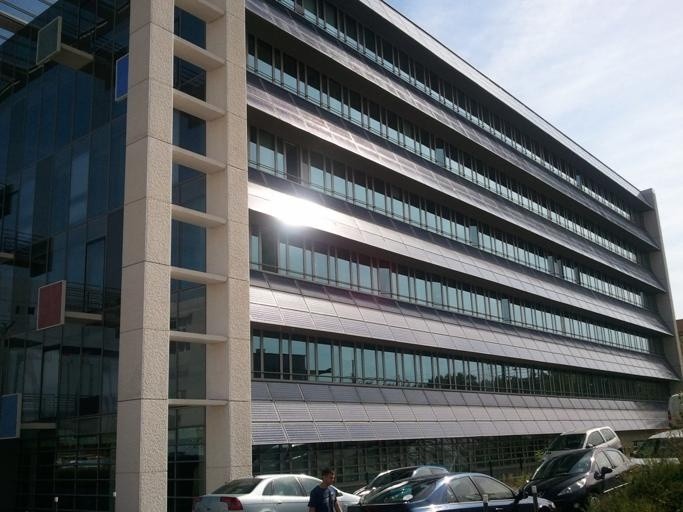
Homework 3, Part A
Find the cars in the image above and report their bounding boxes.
[192,473,361,512]
[542,425,624,461]
[352,465,448,496]
[513,448,645,512]
[632,426,682,472]
[347,471,558,512]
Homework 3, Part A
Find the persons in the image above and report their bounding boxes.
[308,467,343,512]
